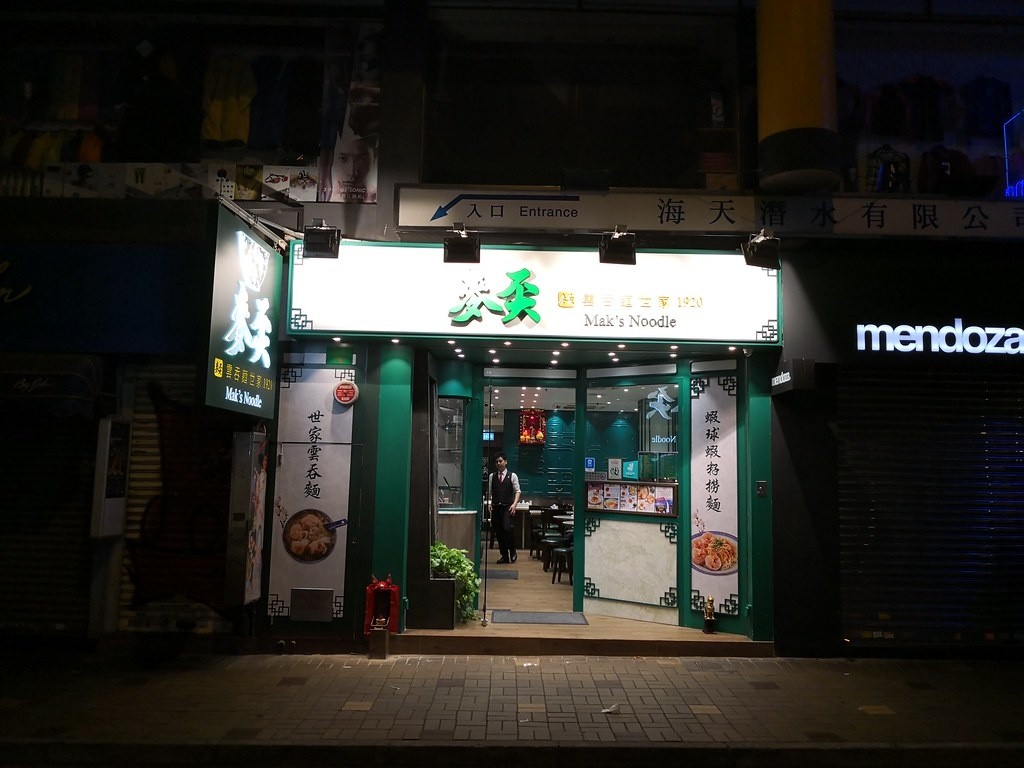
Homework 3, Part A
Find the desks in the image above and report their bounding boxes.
[551,514,575,538]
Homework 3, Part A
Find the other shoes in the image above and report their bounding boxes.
[497,555,509,563]
[510,549,517,562]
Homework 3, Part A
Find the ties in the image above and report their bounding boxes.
[499,472,504,483]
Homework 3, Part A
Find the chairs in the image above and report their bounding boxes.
[527,512,561,563]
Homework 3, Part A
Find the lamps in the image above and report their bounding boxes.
[302,218,342,257]
[598,225,637,265]
[739,228,783,271]
[442,222,481,264]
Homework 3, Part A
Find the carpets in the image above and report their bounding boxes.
[480,569,519,579]
[491,610,589,625]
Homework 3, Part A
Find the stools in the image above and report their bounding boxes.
[543,539,573,586]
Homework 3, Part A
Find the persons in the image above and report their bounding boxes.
[488,453,520,563]
[253,453,264,595]
[332,138,379,205]
